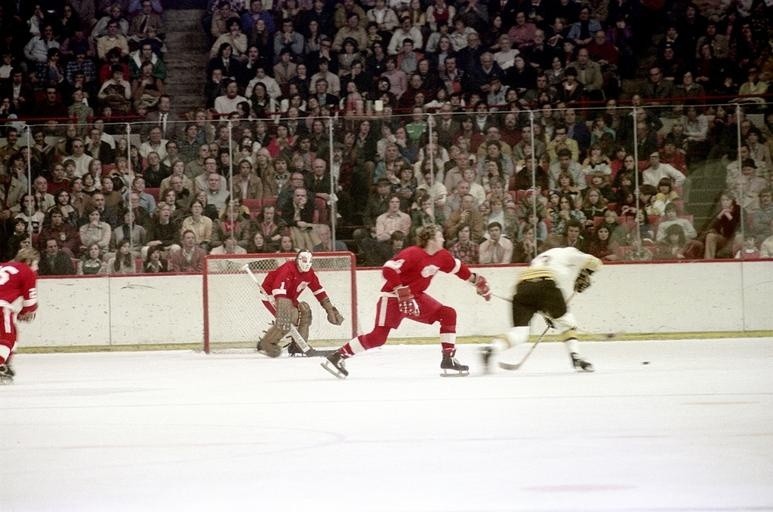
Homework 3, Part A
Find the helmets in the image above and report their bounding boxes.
[14,246,41,265]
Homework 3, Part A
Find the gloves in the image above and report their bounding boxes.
[470,273,491,302]
[574,269,590,293]
[394,285,420,316]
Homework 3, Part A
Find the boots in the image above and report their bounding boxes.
[480,343,492,369]
[440,348,468,371]
[0,363,14,378]
[570,352,592,369]
[325,349,348,376]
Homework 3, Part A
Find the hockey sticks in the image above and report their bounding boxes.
[240,264,337,356]
[497,289,576,369]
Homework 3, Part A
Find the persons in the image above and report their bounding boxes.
[478,245,606,372]
[327,222,492,377]
[252,247,344,357]
[0,0,772,274]
[0,247,41,377]
